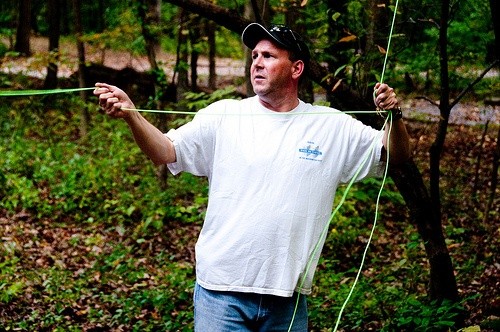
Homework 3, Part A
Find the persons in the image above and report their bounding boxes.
[93,22,409,331]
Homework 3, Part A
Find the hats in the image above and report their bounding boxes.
[241,23,310,63]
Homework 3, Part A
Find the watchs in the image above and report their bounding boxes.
[377,106,403,121]
